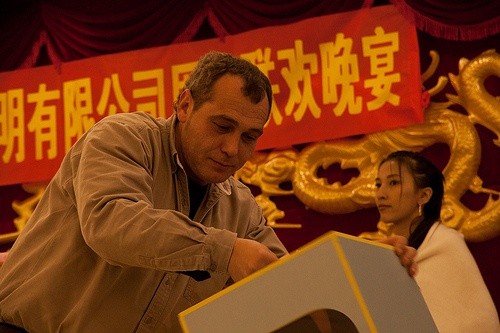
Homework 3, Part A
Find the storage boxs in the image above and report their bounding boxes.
[177,230,440,333]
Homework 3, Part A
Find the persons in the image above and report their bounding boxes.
[371,151,500,333]
[1,51,419,333]
